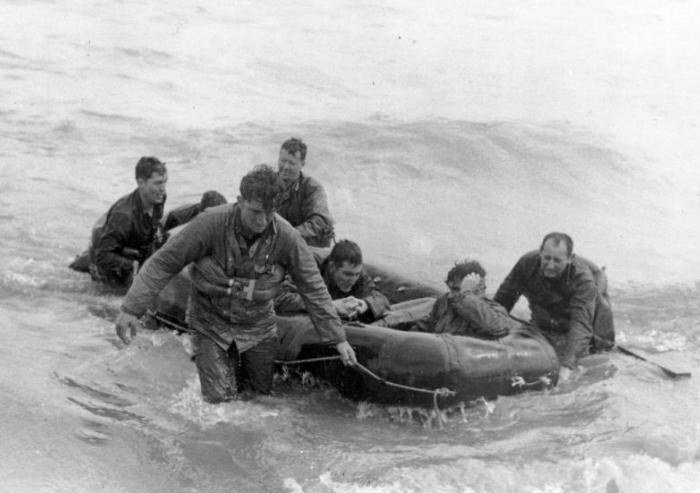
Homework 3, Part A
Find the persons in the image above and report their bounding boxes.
[66,154,170,294]
[404,257,515,339]
[489,230,616,390]
[270,238,391,324]
[272,137,334,267]
[159,188,231,245]
[113,164,359,407]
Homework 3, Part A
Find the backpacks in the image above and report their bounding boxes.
[579,257,615,354]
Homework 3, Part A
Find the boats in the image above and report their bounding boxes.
[125,244,561,412]
